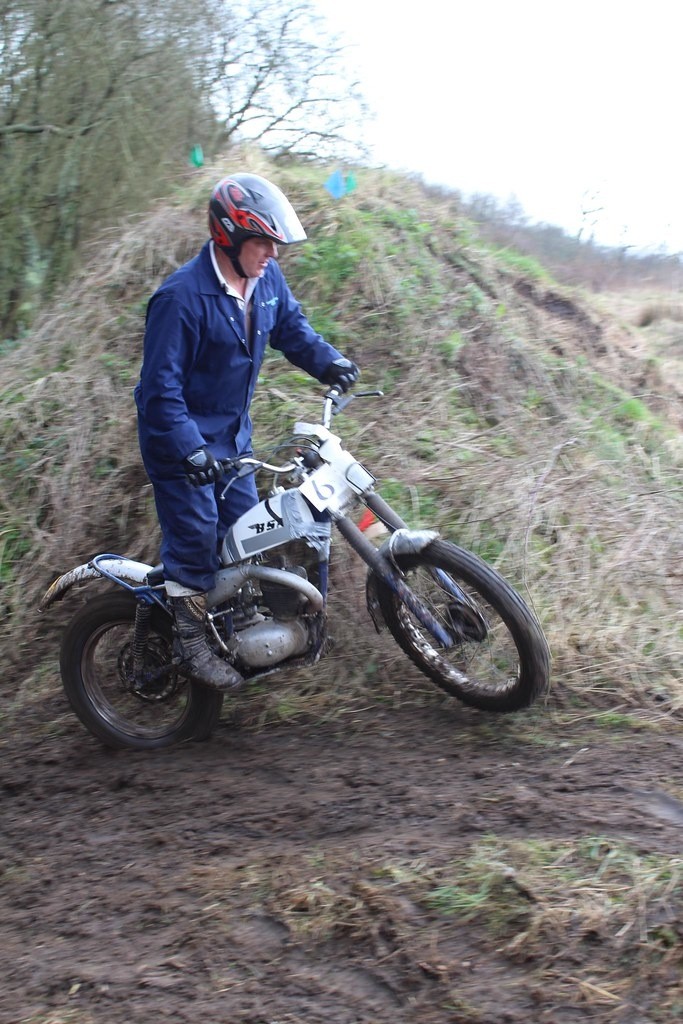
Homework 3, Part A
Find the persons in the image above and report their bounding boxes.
[133,173,362,694]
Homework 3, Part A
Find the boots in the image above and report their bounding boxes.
[164,580,244,692]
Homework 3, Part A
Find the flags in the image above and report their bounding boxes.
[188,143,204,169]
[322,169,359,200]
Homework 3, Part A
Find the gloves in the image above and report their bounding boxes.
[318,356,361,394]
[181,447,225,487]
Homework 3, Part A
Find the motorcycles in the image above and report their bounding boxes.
[35,381,550,754]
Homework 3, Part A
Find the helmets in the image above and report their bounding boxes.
[209,174,307,257]
